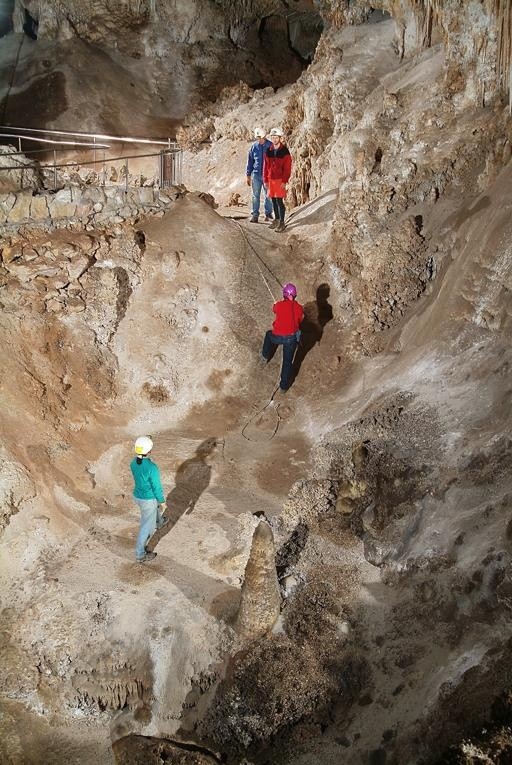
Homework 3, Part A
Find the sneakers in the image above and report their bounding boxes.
[137,553,157,562]
[279,388,288,393]
[157,517,169,530]
[250,215,286,232]
[259,352,268,364]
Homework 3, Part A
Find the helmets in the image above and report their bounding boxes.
[283,283,296,296]
[134,437,153,454]
[254,127,283,138]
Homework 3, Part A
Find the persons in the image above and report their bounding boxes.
[259,283,304,394]
[262,128,292,232]
[246,127,273,222]
[130,436,169,563]
